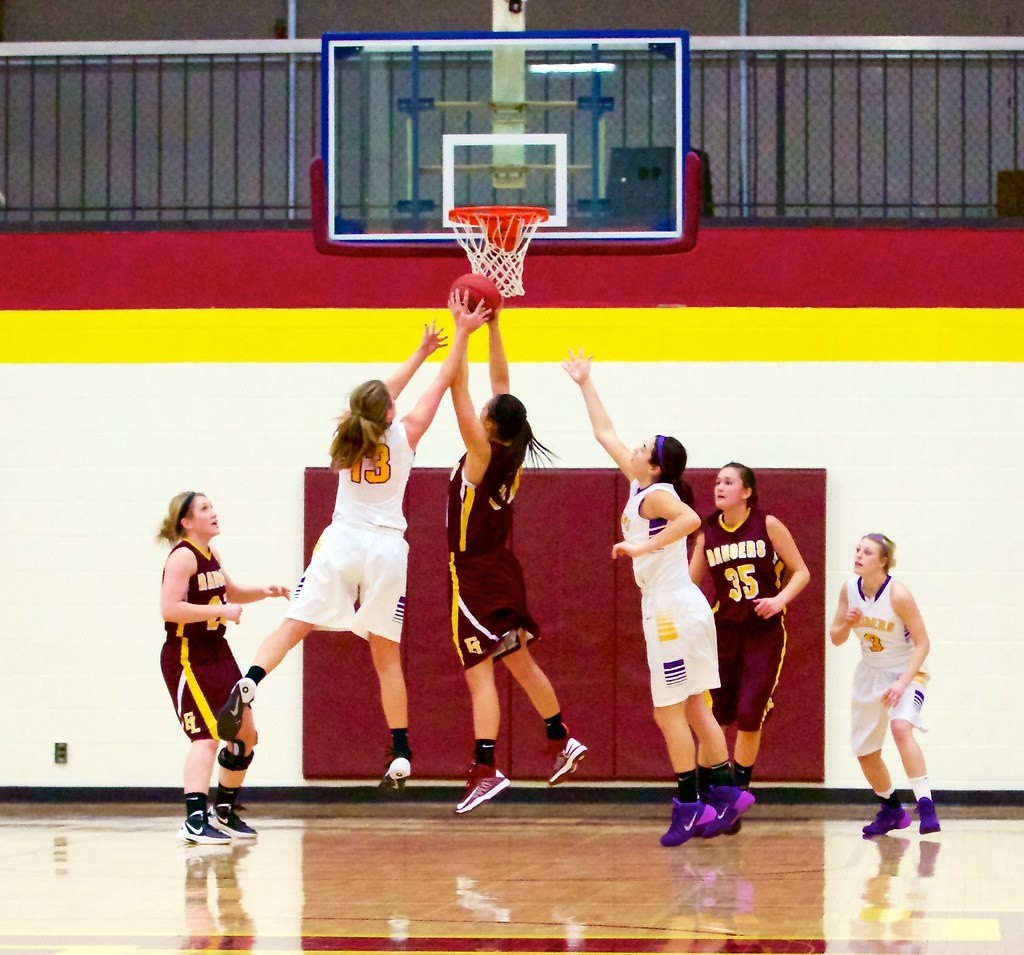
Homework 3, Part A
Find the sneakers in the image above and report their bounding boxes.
[185,845,233,872]
[455,759,511,813]
[660,798,717,846]
[547,736,588,786]
[913,798,940,834]
[206,805,258,838]
[862,804,912,834]
[378,744,410,798]
[217,678,258,740]
[178,818,232,845]
[700,786,755,839]
[233,838,258,859]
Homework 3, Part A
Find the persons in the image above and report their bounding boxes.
[217,294,493,797]
[690,463,811,837]
[563,347,759,847]
[161,490,294,846]
[445,287,591,814]
[829,533,943,835]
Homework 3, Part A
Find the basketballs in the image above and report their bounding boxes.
[451,273,500,322]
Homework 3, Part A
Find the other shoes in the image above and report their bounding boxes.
[725,818,741,836]
